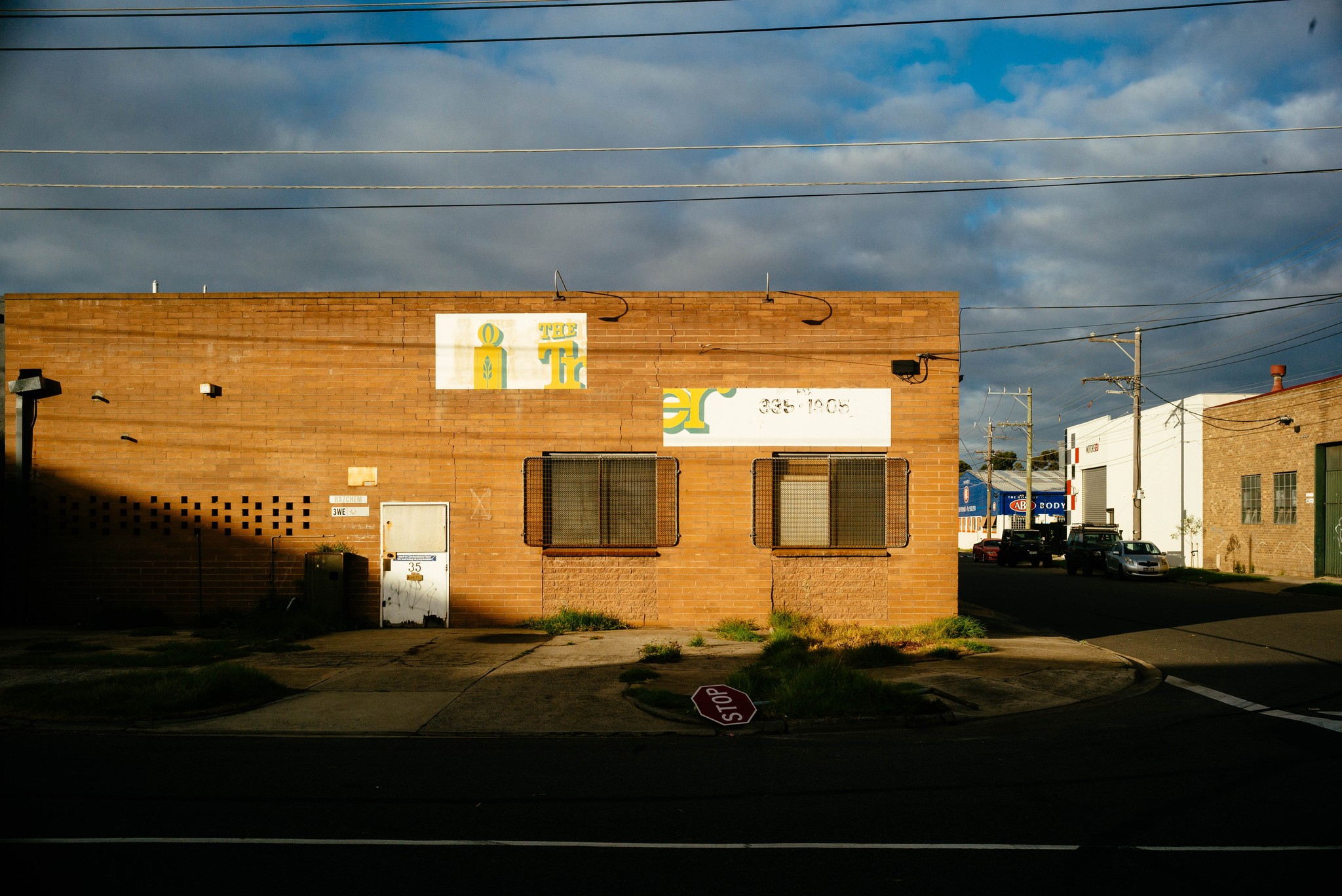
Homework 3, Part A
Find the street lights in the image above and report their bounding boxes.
[7,375,46,469]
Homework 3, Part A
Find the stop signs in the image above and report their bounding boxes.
[691,685,757,726]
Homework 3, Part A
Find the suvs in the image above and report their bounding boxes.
[997,529,1053,568]
[1064,523,1128,578]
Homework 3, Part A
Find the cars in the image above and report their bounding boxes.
[973,538,1002,563]
[1106,541,1169,582]
